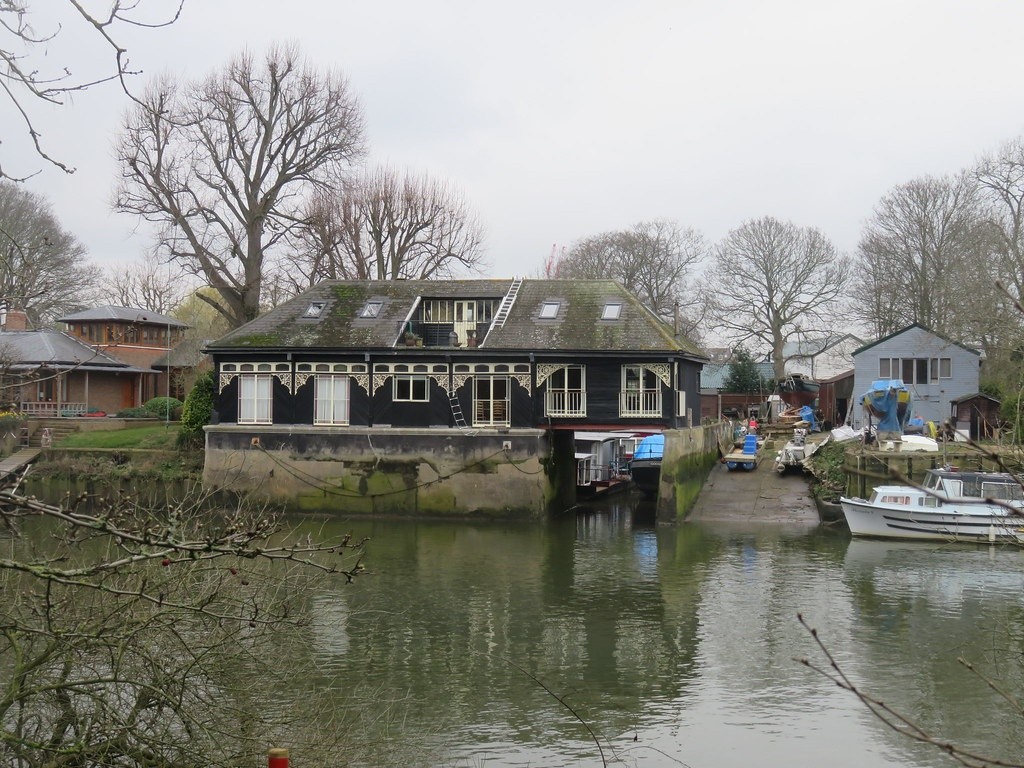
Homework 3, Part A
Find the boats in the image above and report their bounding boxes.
[860,380,909,420]
[777,373,820,406]
[628,435,664,494]
[840,465,1024,545]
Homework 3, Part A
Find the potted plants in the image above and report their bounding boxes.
[405,332,418,346]
[415,337,423,346]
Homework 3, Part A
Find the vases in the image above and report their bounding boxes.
[467,339,478,347]
